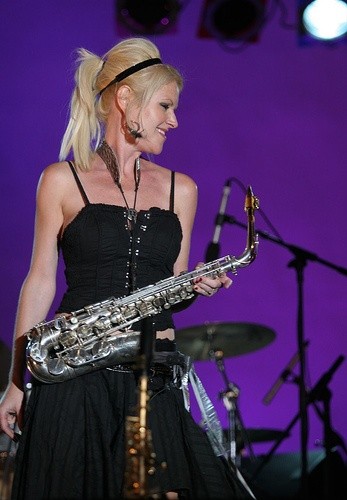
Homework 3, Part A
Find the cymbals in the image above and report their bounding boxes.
[174,319,278,361]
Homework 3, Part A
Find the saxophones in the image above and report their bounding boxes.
[23,183,260,383]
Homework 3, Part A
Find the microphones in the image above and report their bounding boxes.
[263,342,309,404]
[206,181,230,263]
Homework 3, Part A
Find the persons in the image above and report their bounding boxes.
[0,39,249,500]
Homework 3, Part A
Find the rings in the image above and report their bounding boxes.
[208,288,216,295]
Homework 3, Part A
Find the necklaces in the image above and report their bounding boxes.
[95,140,141,231]
